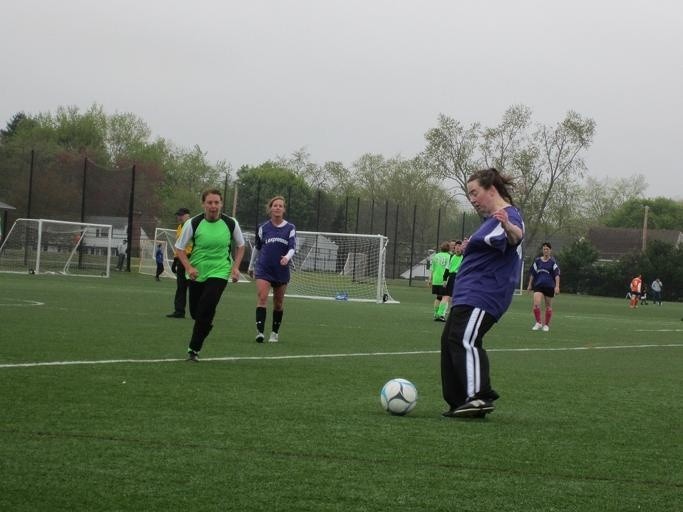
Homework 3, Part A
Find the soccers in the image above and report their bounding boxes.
[380,378,418,414]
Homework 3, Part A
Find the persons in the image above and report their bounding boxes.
[72,235,79,256]
[526,242,560,332]
[650,277,663,306]
[155,244,164,280]
[115,239,127,270]
[247,196,298,344]
[175,189,245,361]
[628,273,641,309]
[438,167,526,418]
[165,207,193,319]
[425,237,465,323]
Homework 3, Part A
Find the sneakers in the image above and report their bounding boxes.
[442,398,494,418]
[187,350,199,362]
[256,331,278,342]
[532,322,549,331]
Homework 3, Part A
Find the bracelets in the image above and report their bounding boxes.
[505,221,512,232]
